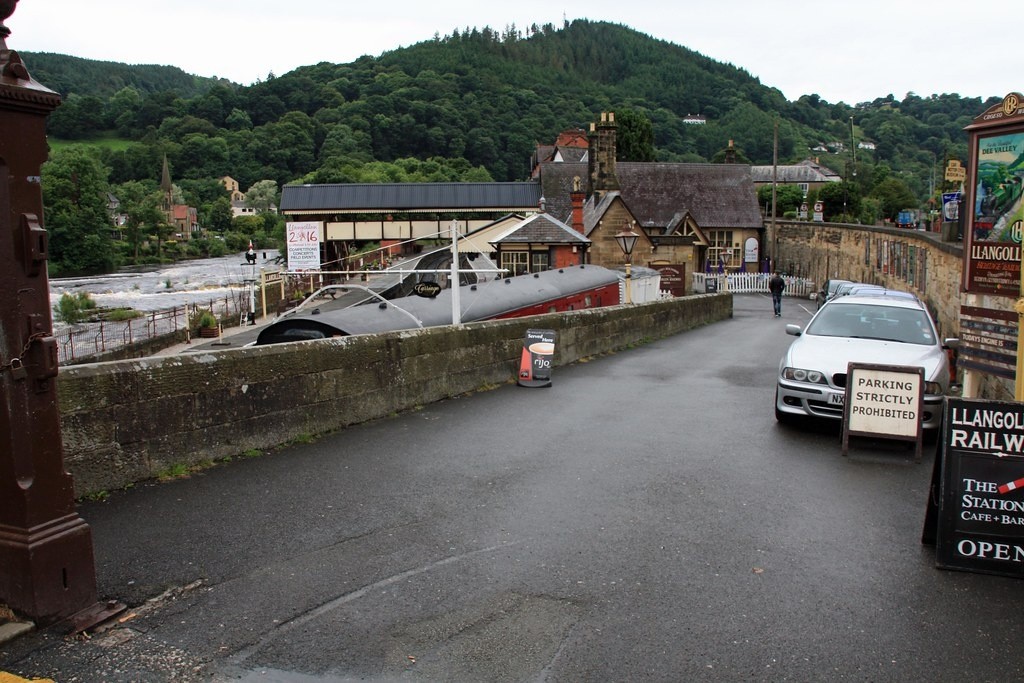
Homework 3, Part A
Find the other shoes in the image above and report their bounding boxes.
[775,313,781,317]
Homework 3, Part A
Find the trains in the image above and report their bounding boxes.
[253,257,661,346]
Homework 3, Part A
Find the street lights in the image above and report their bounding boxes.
[614,219,641,304]
[718,243,734,291]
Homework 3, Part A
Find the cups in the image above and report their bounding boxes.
[529,342,555,381]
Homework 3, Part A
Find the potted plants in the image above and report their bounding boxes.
[200,310,219,337]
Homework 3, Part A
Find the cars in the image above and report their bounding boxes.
[810,279,917,311]
[774,294,960,442]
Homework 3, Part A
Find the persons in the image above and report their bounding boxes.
[769,271,785,317]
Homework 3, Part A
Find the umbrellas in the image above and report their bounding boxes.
[706,259,711,273]
[740,258,746,273]
[718,261,724,273]
[764,257,768,273]
